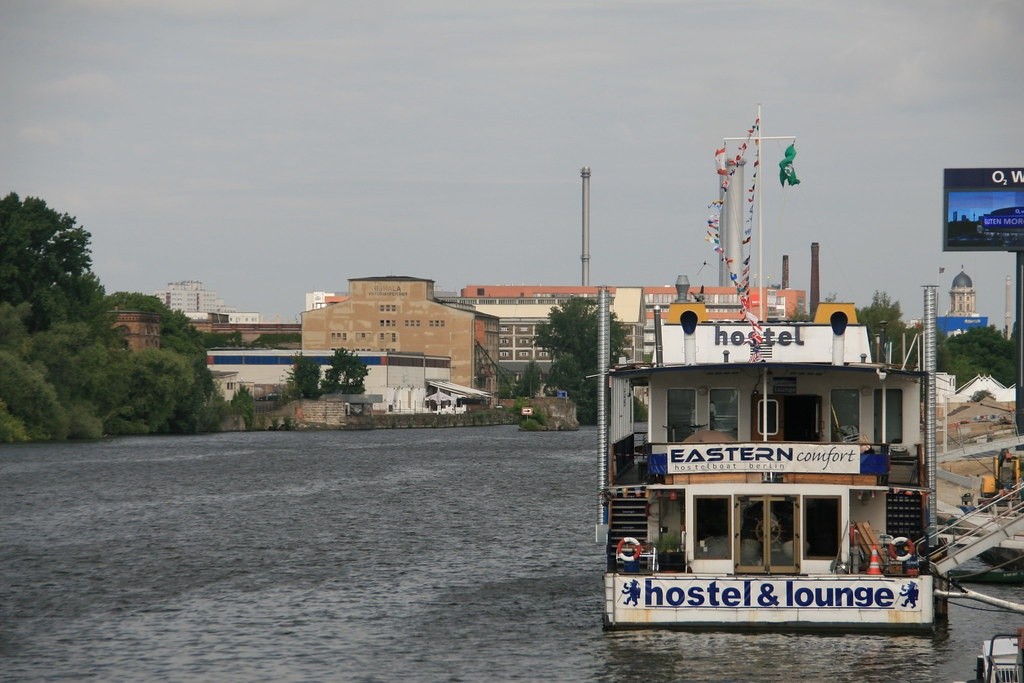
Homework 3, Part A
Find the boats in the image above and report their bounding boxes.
[585,103,951,637]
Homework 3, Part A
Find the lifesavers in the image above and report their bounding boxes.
[617,537,641,562]
[889,537,914,561]
